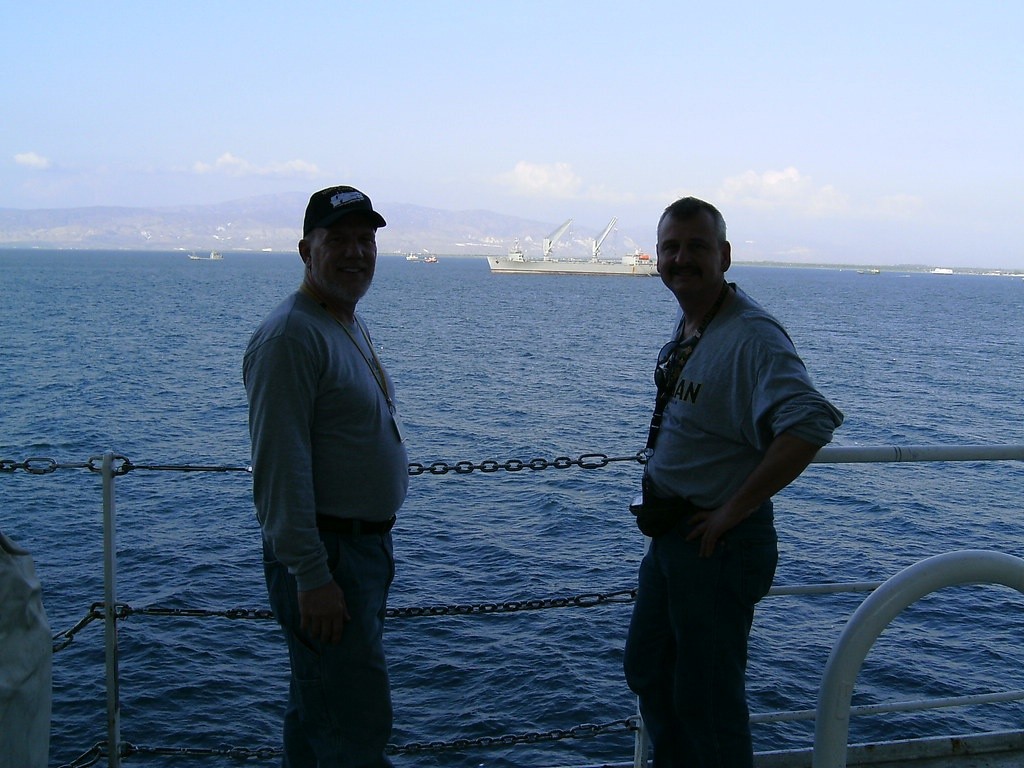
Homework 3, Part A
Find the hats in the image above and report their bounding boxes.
[304,187,387,244]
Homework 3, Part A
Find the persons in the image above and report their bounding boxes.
[242,186,410,768]
[622,197,844,768]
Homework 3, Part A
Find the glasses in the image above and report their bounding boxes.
[654,340,681,392]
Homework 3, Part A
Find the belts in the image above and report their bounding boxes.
[315,511,397,533]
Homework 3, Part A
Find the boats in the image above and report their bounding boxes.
[638,254,649,260]
[485,216,661,277]
[931,267,953,275]
[405,252,419,261]
[857,268,881,274]
[187,250,225,261]
[422,255,439,263]
[262,248,272,252]
